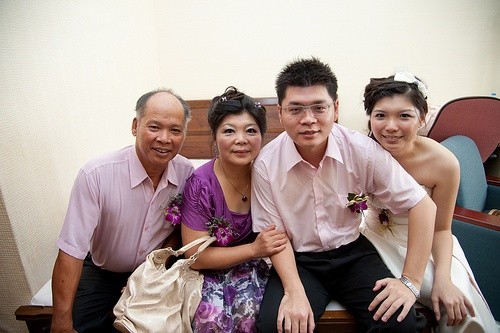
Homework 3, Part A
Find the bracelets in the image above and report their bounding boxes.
[399,276,422,300]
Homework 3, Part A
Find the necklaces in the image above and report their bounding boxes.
[220,164,251,202]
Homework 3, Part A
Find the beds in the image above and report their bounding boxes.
[16,98,435,332]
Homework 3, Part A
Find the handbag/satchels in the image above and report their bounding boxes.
[113,235,218,332]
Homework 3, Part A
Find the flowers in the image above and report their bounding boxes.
[206,215,234,247]
[346,192,372,218]
[373,207,398,240]
[161,191,183,227]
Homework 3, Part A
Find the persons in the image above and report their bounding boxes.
[249,57,429,333]
[50,88,196,333]
[357,71,500,333]
[181,84,289,333]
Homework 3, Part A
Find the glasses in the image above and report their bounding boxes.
[280,100,336,115]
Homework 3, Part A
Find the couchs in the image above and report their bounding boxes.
[439,134,500,296]
